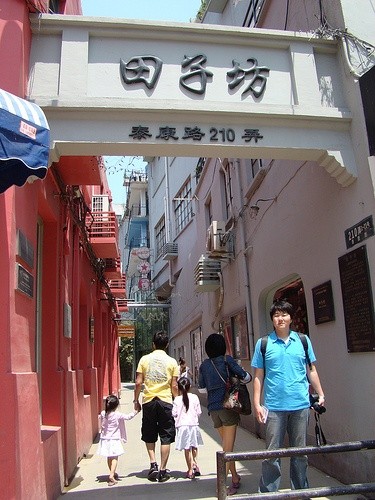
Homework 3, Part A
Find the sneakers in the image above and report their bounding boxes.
[148,462,159,479]
[158,469,170,482]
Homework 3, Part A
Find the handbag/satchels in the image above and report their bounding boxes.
[223,355,252,415]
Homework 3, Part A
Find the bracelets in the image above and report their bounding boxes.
[133,400,138,402]
[319,395,325,400]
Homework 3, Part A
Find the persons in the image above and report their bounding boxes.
[98,330,204,486]
[250,301,325,500]
[198,333,253,495]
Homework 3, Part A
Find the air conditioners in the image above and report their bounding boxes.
[91,194,110,237]
[206,221,227,259]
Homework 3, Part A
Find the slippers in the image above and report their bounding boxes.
[186,471,194,479]
[226,485,237,496]
[114,473,118,479]
[108,480,115,486]
[193,465,200,476]
[232,475,241,488]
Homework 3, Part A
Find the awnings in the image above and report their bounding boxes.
[0,86,50,191]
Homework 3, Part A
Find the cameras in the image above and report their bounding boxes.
[310,393,326,415]
